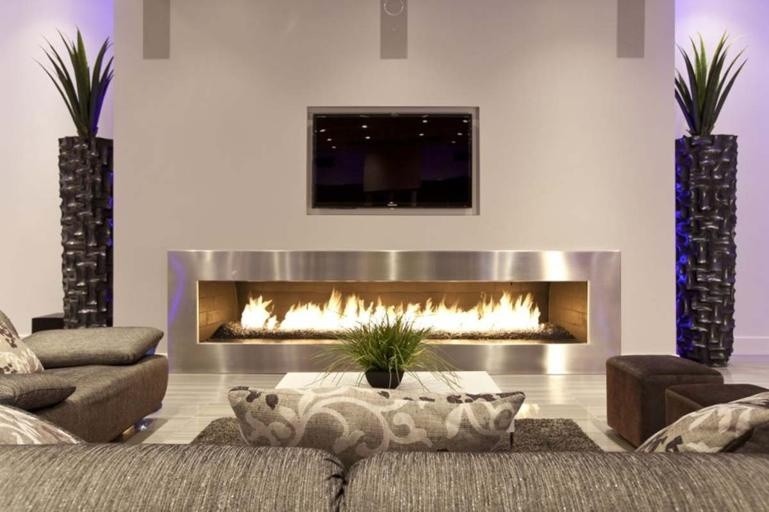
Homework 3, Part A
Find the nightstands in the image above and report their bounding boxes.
[32,312,64,334]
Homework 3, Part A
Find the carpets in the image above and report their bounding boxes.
[189,418,605,454]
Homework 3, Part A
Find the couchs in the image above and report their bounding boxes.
[30,352,169,444]
[0,440,768,512]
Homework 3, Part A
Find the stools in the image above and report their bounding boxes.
[664,384,769,426]
[606,355,724,449]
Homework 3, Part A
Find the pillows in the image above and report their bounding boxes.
[0,318,45,375]
[227,386,525,477]
[0,403,89,446]
[23,325,165,368]
[633,391,768,452]
[0,372,76,409]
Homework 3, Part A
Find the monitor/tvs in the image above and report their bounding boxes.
[312,113,472,208]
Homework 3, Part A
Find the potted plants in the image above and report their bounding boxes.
[310,304,462,394]
[34,24,113,329]
[673,30,749,366]
